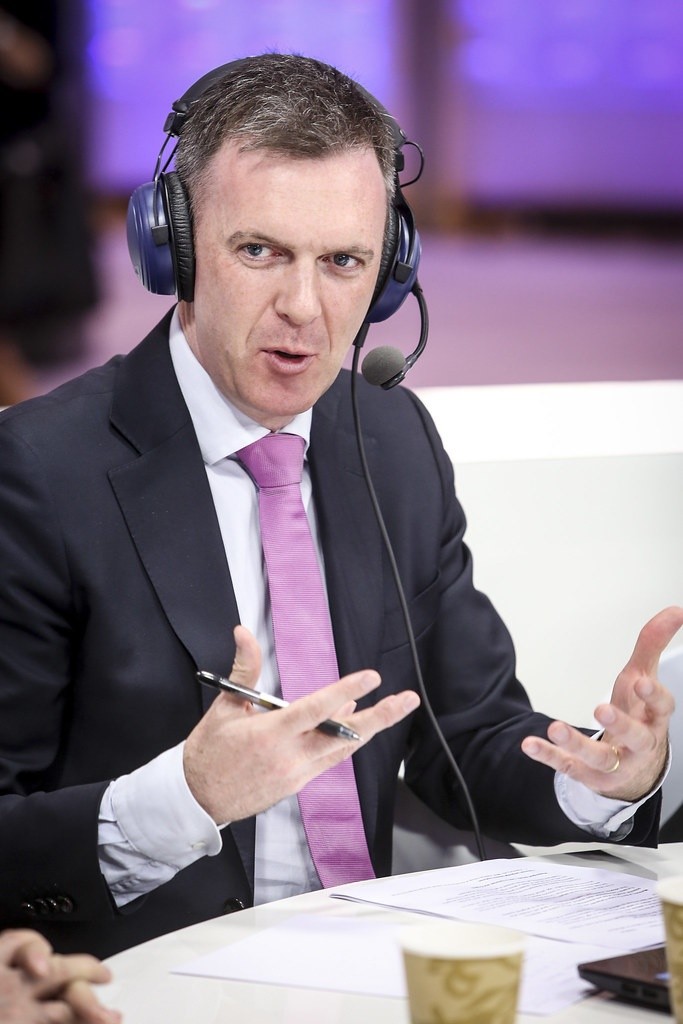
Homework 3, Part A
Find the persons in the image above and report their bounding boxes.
[0,928,128,1023]
[0,51,681,964]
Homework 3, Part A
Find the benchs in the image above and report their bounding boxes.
[411,380,683,729]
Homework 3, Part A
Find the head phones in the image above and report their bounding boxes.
[126,57,424,326]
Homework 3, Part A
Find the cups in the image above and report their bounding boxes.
[656,878,683,1024]
[400,920,527,1024]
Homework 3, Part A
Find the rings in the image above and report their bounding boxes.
[603,746,621,778]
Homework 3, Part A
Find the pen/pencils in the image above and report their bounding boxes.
[194,671,365,743]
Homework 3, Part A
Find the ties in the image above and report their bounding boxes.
[236,432,377,889]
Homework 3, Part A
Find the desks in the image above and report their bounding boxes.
[100,842,683,1024]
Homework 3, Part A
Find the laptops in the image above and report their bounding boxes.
[577,947,672,1009]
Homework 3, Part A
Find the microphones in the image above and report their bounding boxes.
[362,277,429,390]
